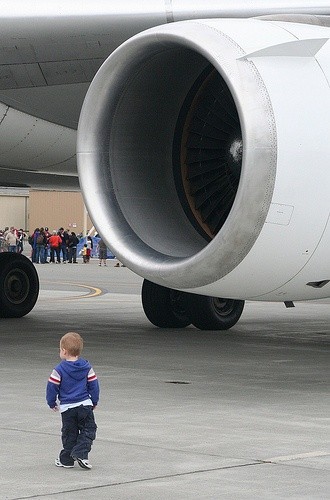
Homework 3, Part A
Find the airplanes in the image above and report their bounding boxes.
[0,2,330,330]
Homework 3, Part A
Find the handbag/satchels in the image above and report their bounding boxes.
[68,241,75,248]
[27,237,34,244]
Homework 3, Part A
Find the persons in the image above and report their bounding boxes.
[28,227,94,264]
[114,259,126,267]
[0,226,28,254]
[46,331,100,469]
[98,238,107,266]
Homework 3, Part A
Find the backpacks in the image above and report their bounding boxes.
[86,248,91,256]
[37,234,45,245]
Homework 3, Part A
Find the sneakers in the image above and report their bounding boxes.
[70,452,93,470]
[54,457,74,468]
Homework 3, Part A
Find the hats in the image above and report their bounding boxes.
[9,226,15,231]
[58,228,64,230]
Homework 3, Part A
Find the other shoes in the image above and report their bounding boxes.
[45,261,49,263]
[63,261,65,263]
[68,261,72,263]
[104,263,106,265]
[36,262,39,264]
[55,261,59,264]
[50,261,52,263]
[73,261,78,264]
[97,264,101,266]
[114,263,126,267]
